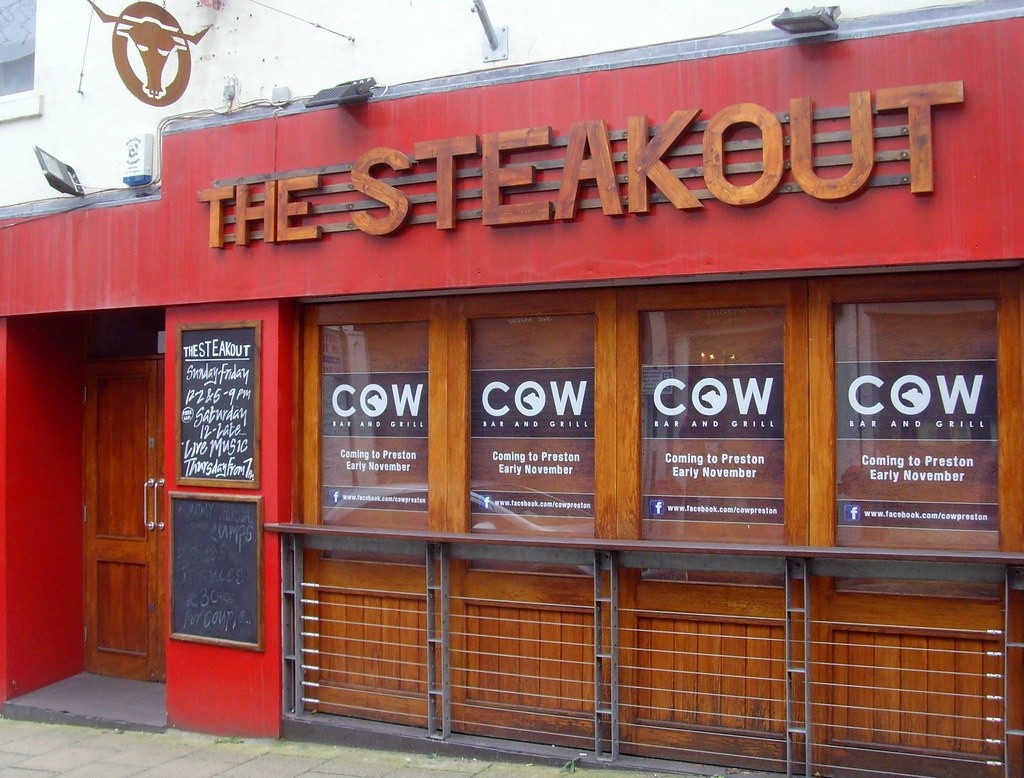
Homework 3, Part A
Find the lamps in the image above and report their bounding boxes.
[33,146,85,197]
[770,5,843,34]
[304,77,378,108]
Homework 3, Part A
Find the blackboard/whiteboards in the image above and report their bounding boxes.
[175,320,261,489]
[169,490,264,652]
